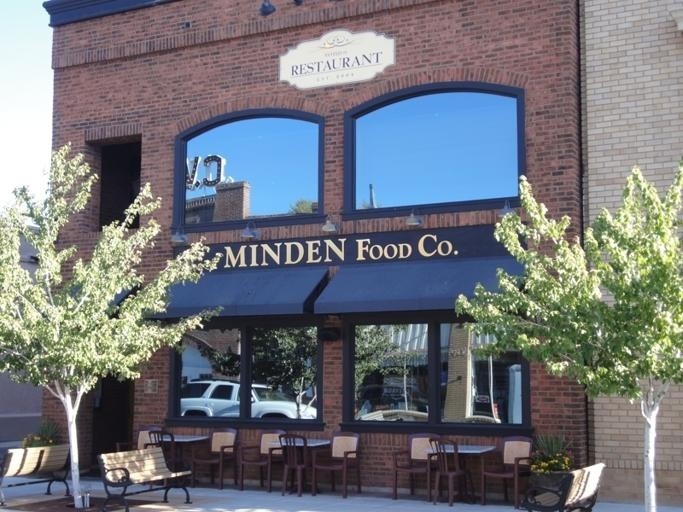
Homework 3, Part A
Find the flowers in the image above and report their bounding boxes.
[528,433,578,476]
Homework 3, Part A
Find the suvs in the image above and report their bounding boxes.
[178,379,317,420]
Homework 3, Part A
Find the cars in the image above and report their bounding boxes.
[354,383,502,425]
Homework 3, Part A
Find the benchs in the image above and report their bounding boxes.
[1,444,71,506]
[96,447,193,512]
[521,461,606,511]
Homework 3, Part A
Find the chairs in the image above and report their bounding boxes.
[392,433,534,512]
[116,424,362,499]
